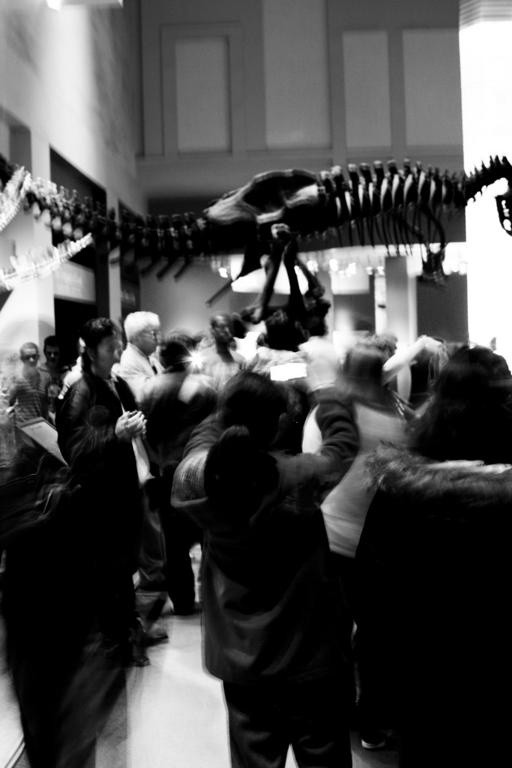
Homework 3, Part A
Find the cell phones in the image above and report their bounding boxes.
[269,360,309,382]
